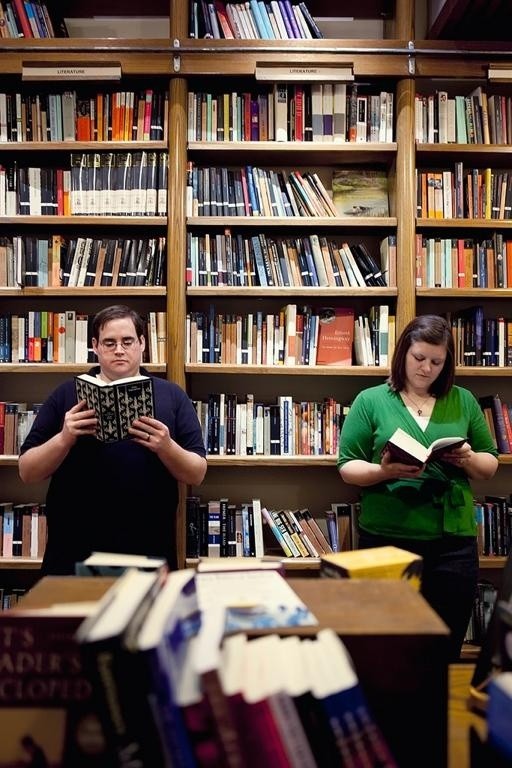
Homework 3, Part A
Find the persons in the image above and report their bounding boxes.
[337,317,499,662]
[18,306,208,576]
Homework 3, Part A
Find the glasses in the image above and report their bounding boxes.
[100,338,139,351]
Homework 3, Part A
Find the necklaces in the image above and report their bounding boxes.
[403,391,432,416]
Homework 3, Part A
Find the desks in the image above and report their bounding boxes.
[2,576,446,693]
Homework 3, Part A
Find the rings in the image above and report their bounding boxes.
[146,434,151,441]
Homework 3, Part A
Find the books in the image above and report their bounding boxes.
[1,402,49,608]
[0,88,167,142]
[76,374,153,441]
[195,391,353,456]
[187,496,364,559]
[187,81,396,143]
[2,546,426,768]
[185,161,388,217]
[186,227,396,287]
[0,309,168,365]
[1,234,166,286]
[380,427,467,467]
[416,87,511,640]
[0,1,69,38]
[186,304,396,367]
[2,149,168,217]
[188,0,323,40]
